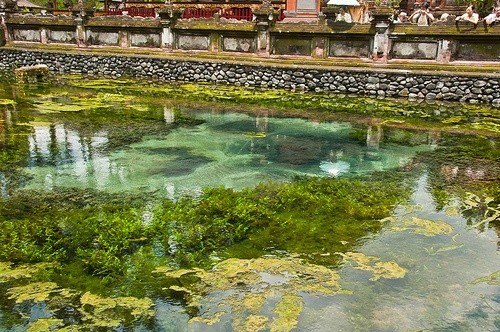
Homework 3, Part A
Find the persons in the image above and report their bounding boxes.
[393,1,435,27]
[483,6,500,25]
[334,6,352,24]
[455,4,479,25]
